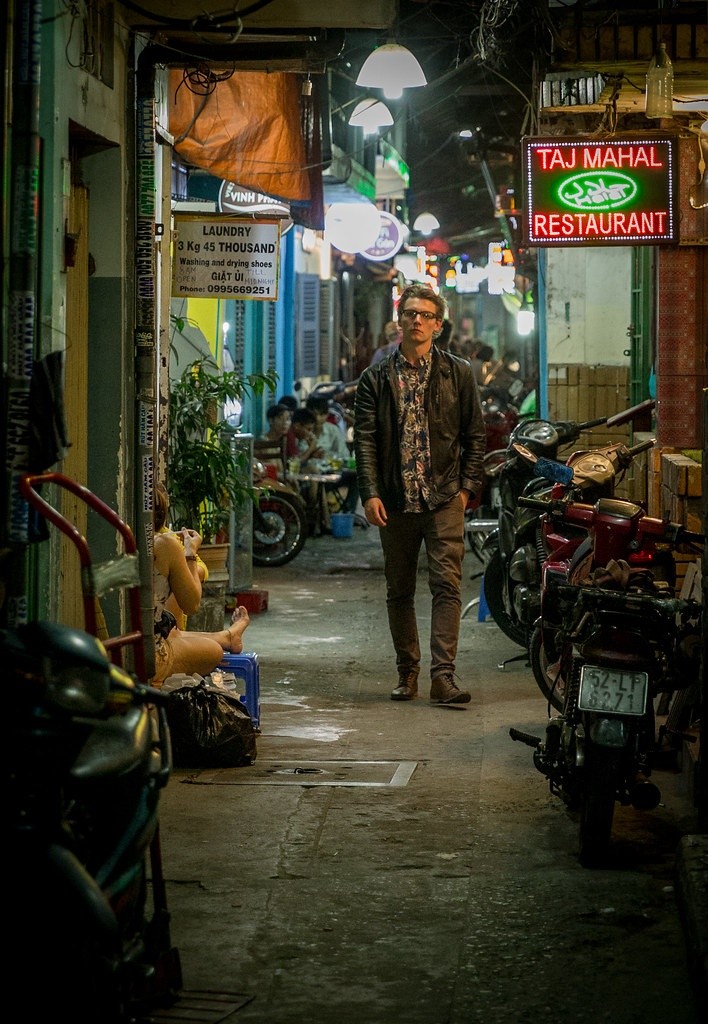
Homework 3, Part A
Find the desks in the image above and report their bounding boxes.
[282,472,370,533]
[466,519,499,579]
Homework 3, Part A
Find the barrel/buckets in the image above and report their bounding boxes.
[331,513,354,539]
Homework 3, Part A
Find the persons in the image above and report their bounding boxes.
[252,396,362,526]
[434,320,538,412]
[354,286,486,704]
[151,483,249,682]
[370,321,403,367]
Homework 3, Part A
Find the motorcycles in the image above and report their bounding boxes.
[0,620,185,1024]
[481,417,706,868]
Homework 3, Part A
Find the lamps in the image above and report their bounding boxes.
[348,93,394,133]
[356,38,428,100]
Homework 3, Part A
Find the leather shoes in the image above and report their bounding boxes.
[391,669,419,699]
[429,672,471,703]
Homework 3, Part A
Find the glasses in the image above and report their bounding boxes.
[400,309,438,321]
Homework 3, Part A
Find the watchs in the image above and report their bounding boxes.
[184,554,198,562]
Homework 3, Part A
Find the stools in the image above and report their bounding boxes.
[217,652,261,728]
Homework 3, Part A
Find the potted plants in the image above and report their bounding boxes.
[157,315,280,582]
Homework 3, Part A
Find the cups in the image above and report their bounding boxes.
[287,457,301,476]
[330,453,344,474]
